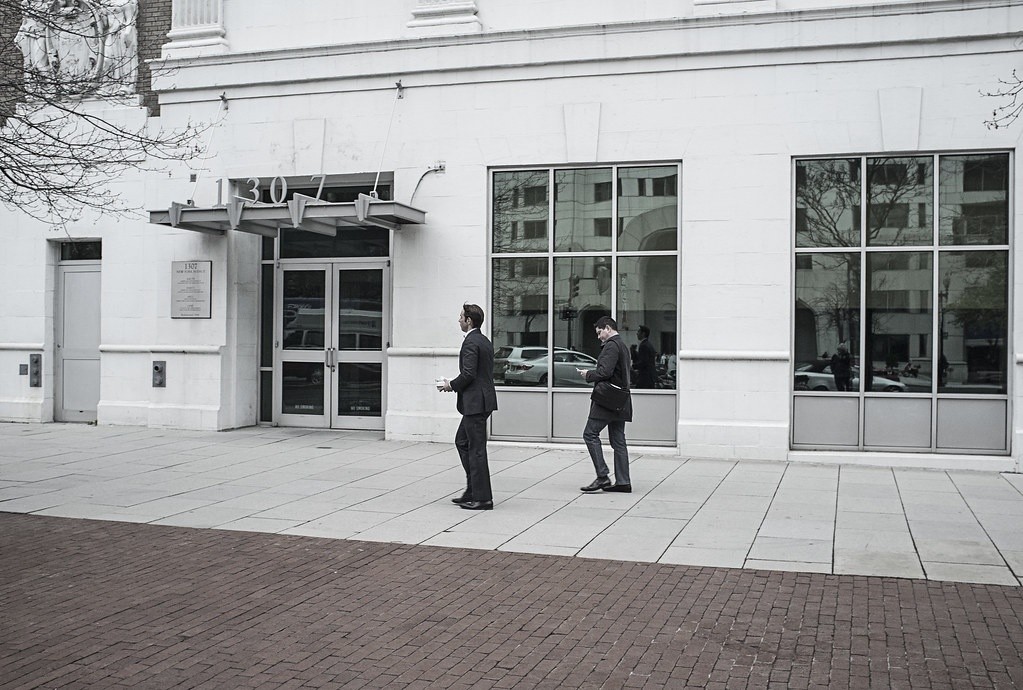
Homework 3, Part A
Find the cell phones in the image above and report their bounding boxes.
[576,368,581,373]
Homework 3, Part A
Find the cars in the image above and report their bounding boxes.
[282,296,382,387]
[793,358,912,392]
[492,343,598,388]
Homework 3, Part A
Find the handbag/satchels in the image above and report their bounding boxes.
[590,380,630,413]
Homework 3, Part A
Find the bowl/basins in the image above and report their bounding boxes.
[435,378,445,390]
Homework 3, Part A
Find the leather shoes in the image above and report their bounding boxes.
[603,484,631,493]
[452,497,473,504]
[580,475,611,491]
[459,499,493,510]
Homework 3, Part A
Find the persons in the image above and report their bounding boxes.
[630,325,676,389]
[580,316,632,493]
[822,343,855,392]
[435,305,498,510]
